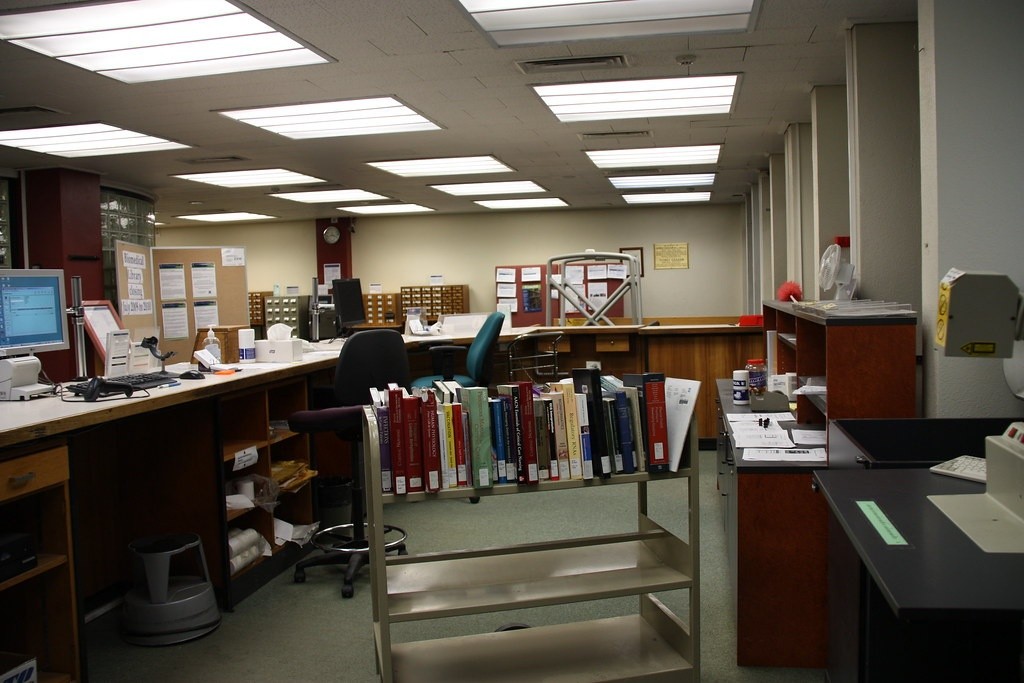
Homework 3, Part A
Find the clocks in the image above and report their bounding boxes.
[324,227,340,245]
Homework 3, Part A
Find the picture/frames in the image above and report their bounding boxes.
[620,247,644,278]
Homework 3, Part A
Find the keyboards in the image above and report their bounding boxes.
[929,455,986,484]
[64,372,177,398]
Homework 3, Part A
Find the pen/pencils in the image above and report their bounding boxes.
[157,382,180,389]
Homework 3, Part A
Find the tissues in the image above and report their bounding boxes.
[254,323,303,363]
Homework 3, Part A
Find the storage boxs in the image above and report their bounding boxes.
[0,651,38,683]
[770,373,797,401]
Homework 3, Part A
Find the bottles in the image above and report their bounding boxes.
[745,359,767,398]
[835,236,850,264]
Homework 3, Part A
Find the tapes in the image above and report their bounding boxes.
[772,390,783,395]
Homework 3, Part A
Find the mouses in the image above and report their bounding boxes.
[179,370,205,379]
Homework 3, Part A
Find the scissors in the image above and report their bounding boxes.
[200,370,236,375]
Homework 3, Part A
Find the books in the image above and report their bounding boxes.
[368,368,702,497]
[193,349,243,372]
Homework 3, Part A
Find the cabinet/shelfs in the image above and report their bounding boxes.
[248,285,470,342]
[761,299,917,465]
[132,374,311,614]
[363,372,704,683]
[715,378,831,668]
[0,433,80,683]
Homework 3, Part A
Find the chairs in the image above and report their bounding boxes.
[410,312,505,505]
[288,330,411,599]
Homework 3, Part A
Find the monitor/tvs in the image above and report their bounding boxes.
[332,278,366,325]
[0,269,70,357]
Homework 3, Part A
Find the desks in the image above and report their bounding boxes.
[809,462,1024,683]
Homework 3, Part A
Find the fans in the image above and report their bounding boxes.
[817,244,858,305]
